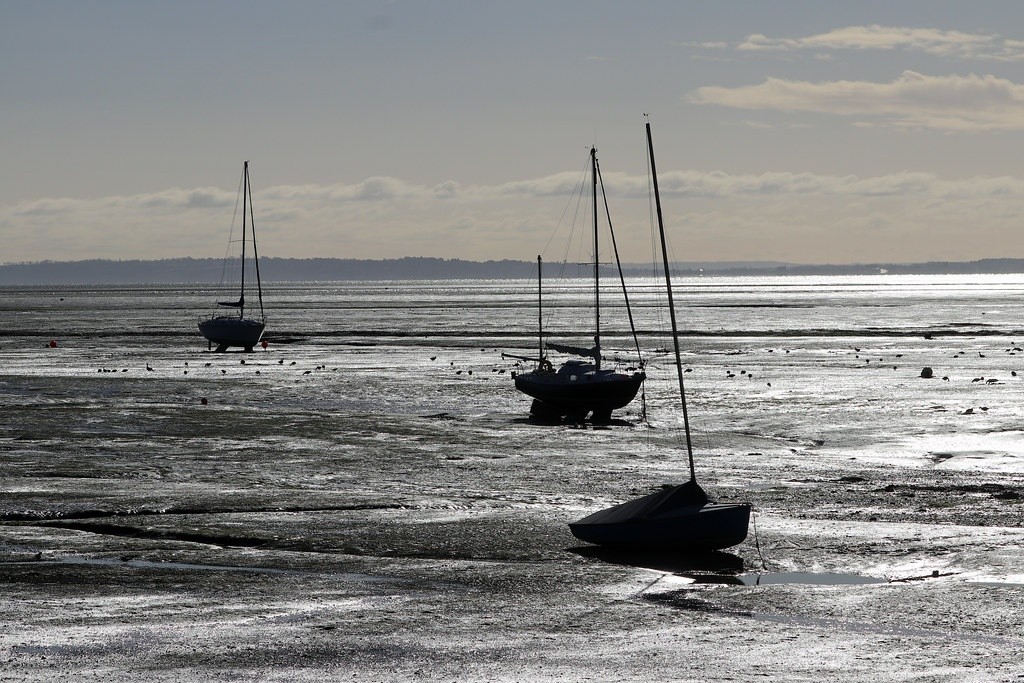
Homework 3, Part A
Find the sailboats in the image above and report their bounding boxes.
[198,158,269,352]
[566,116,756,554]
[499,144,647,418]
